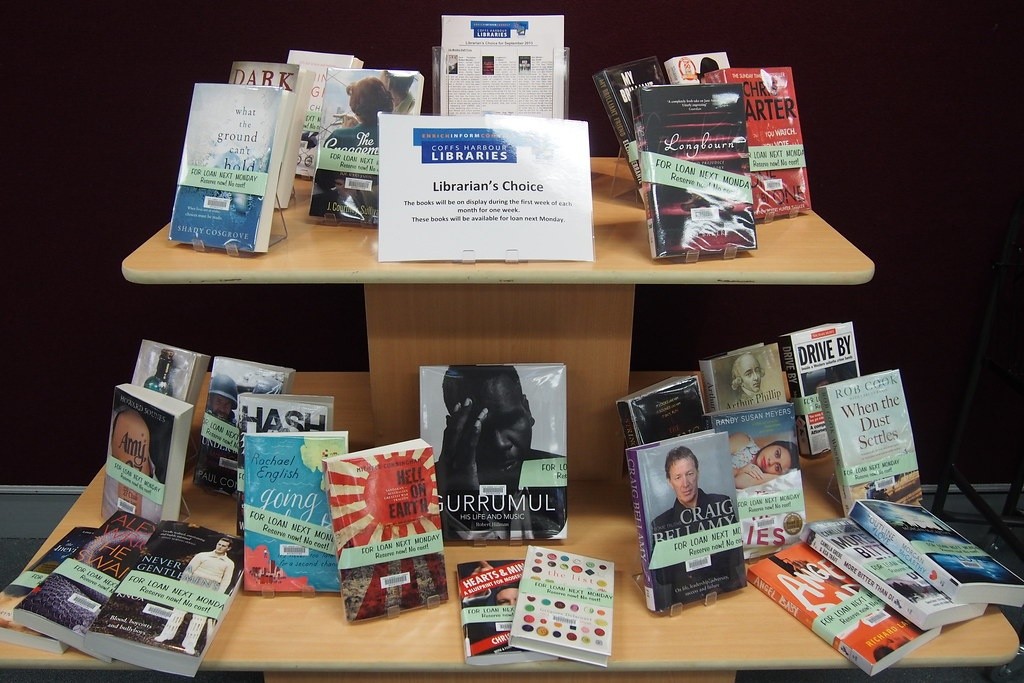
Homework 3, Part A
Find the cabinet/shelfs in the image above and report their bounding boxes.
[0,157,1020,683]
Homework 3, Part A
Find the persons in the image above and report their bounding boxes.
[732,353,765,398]
[330,71,415,129]
[728,432,800,489]
[652,446,743,604]
[311,70,394,226]
[155,539,234,654]
[461,561,519,657]
[434,366,566,540]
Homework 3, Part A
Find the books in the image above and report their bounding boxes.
[0,15,1024,683]
